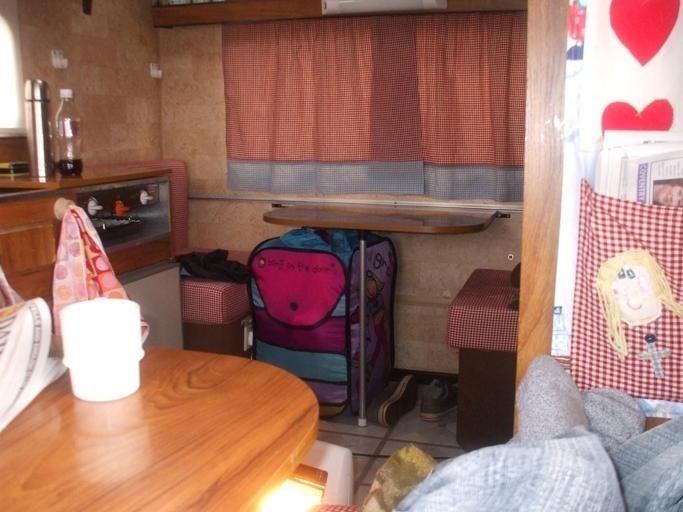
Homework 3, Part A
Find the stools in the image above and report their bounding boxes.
[443,265,520,453]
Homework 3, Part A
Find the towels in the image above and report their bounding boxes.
[49,205,150,348]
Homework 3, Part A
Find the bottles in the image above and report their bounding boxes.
[53,87,84,178]
[21,79,53,179]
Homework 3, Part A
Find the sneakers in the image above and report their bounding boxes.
[419,378,457,422]
[366,374,417,428]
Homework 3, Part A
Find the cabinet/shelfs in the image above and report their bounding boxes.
[0,165,175,328]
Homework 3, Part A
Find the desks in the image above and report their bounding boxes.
[260,201,500,430]
[0,347,321,511]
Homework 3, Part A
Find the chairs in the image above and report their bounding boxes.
[115,159,256,323]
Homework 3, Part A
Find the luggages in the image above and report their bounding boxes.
[245,230,397,421]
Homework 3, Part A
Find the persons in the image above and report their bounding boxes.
[652,176,682,209]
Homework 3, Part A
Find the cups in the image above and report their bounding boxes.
[70,397,152,486]
[59,296,140,404]
